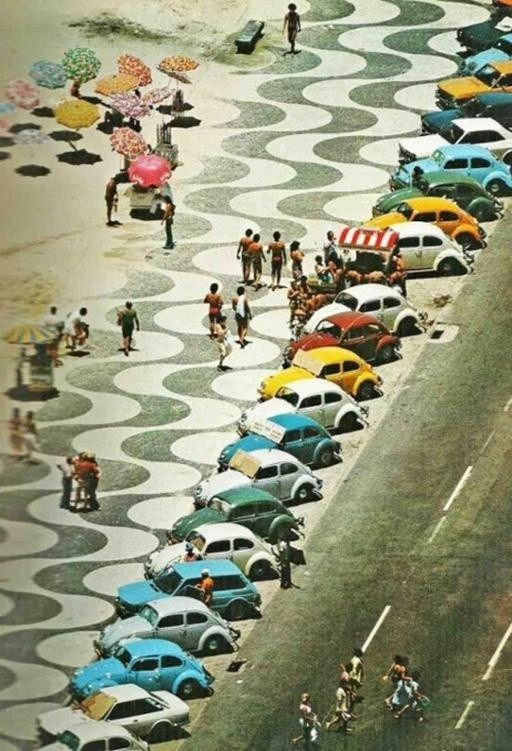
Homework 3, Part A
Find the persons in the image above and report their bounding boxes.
[183,543,201,561]
[8,306,101,514]
[106,177,121,224]
[203,229,410,369]
[291,646,430,749]
[194,569,214,607]
[282,3,301,53]
[279,532,291,590]
[116,301,139,356]
[161,196,175,250]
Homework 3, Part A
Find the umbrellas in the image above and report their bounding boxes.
[1,323,61,344]
[0,46,200,189]
